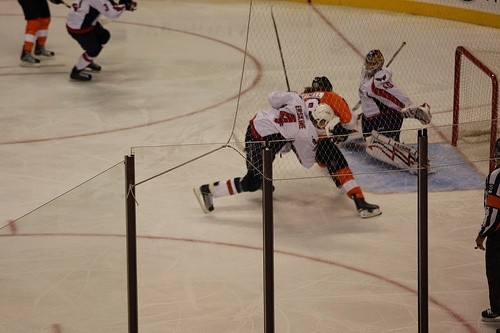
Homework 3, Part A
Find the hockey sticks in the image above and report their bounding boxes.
[351,42,406,112]
[270,4,291,91]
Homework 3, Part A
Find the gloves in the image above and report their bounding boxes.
[119,0,137,12]
[330,122,352,144]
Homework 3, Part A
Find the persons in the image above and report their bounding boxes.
[200,90,333,211]
[475,138,500,318]
[358,50,433,174]
[299,76,380,211]
[17,0,63,63]
[66,0,136,80]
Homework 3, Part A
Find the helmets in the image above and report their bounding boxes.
[360,49,385,79]
[312,76,333,88]
[311,103,334,129]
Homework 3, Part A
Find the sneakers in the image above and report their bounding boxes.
[85,61,102,73]
[193,181,219,213]
[481,308,500,322]
[69,65,92,81]
[351,194,382,217]
[19,45,41,66]
[33,40,55,60]
